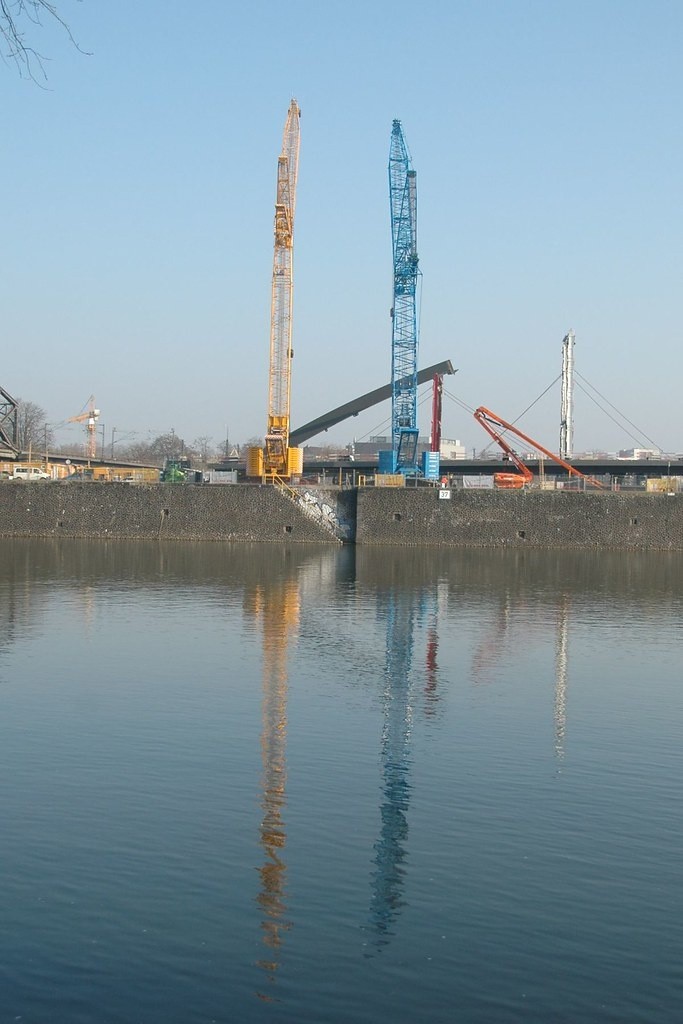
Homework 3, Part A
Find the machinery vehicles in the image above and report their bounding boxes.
[246,95,305,485]
[373,118,441,487]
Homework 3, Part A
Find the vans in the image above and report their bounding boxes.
[13,466,51,481]
[65,470,94,481]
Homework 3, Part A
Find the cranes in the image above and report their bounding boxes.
[67,394,101,458]
